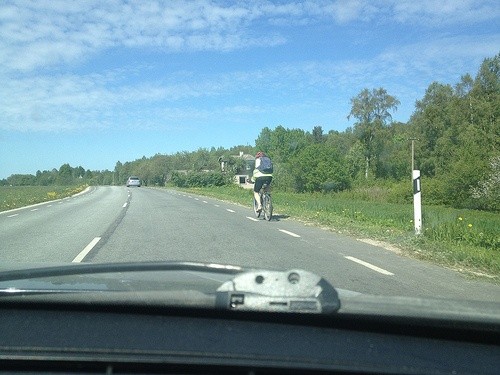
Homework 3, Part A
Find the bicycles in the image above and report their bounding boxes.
[247,179,272,221]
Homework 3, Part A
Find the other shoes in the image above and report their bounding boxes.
[257,206,262,213]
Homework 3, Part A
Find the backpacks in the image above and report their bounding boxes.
[256,156,273,174]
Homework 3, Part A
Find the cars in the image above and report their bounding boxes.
[126,176,140,187]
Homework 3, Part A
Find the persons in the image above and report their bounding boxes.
[249,151,274,212]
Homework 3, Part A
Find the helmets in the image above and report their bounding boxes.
[256,152,265,158]
